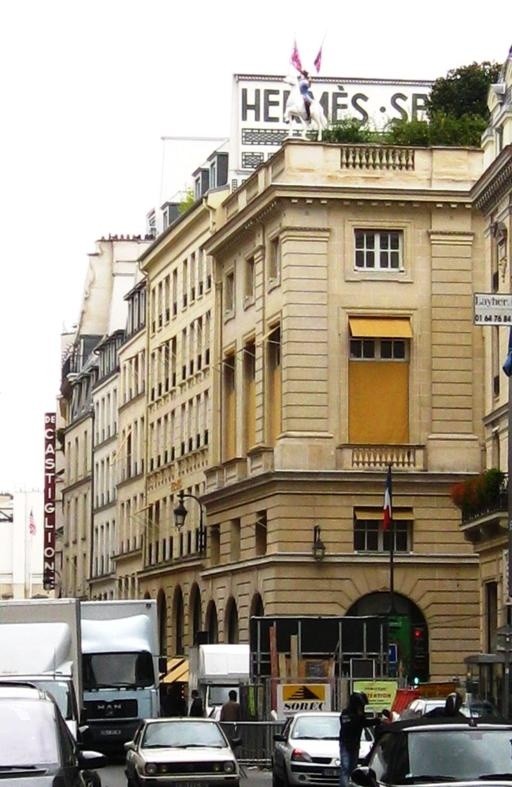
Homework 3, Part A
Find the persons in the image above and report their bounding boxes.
[219,691,241,722]
[189,689,202,716]
[292,70,312,120]
[340,692,381,786]
[423,692,466,719]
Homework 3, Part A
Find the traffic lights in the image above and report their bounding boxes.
[410,623,428,679]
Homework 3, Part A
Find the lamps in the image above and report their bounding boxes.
[311,524,326,560]
[173,489,203,553]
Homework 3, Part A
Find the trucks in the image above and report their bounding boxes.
[82,598,167,750]
[1,594,90,748]
[187,642,251,721]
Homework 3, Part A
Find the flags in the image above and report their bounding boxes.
[29,511,38,535]
[290,49,302,71]
[314,51,322,73]
[382,473,395,528]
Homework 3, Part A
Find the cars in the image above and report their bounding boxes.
[124,717,242,786]
[347,717,511,786]
[1,678,108,786]
[271,710,377,787]
[397,695,500,721]
